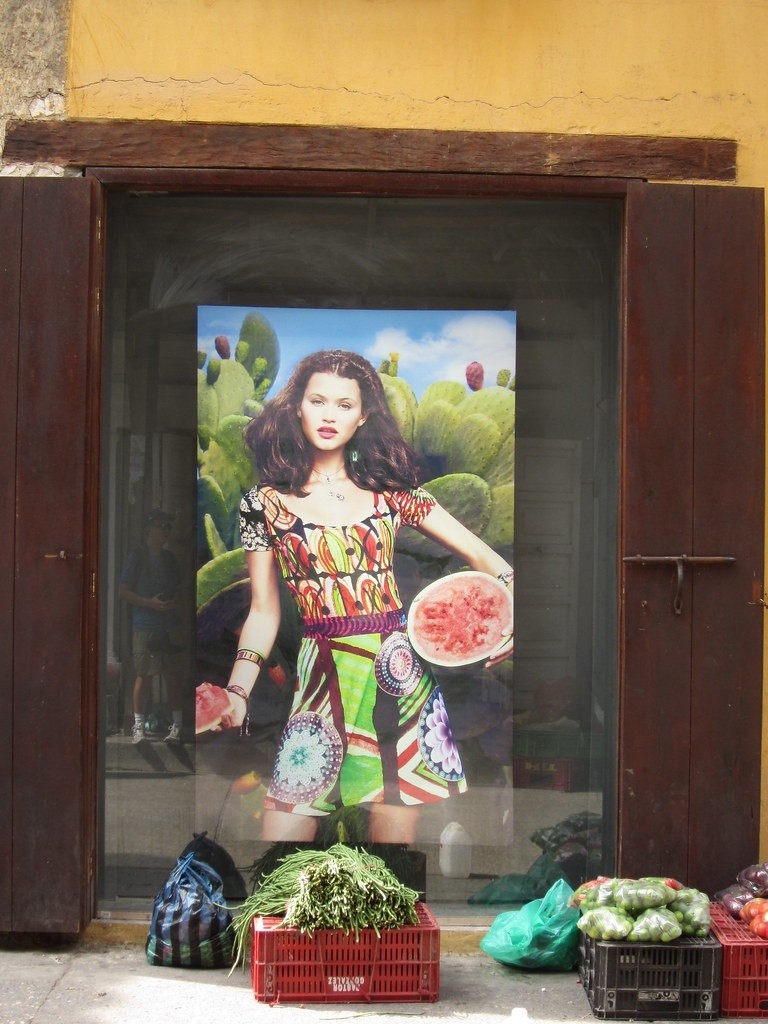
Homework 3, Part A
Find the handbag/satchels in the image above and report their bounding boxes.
[479,878,581,972]
[179,828,248,901]
[468,851,572,903]
[145,850,243,968]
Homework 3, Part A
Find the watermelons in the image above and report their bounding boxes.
[406,571,514,669]
[195,680,233,735]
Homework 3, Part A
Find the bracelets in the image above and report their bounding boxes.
[230,647,266,669]
[226,685,251,737]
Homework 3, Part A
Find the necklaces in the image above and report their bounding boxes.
[312,463,347,501]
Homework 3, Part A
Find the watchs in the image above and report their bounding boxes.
[498,570,514,587]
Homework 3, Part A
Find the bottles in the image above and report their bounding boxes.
[500,1007,536,1024]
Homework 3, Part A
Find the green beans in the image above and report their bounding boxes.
[241,805,418,893]
[212,839,427,982]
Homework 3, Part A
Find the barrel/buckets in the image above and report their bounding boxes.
[439,822,472,878]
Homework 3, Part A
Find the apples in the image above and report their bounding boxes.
[575,877,711,941]
[715,862,768,941]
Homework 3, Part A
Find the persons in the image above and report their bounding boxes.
[210,349,514,843]
[118,507,190,745]
